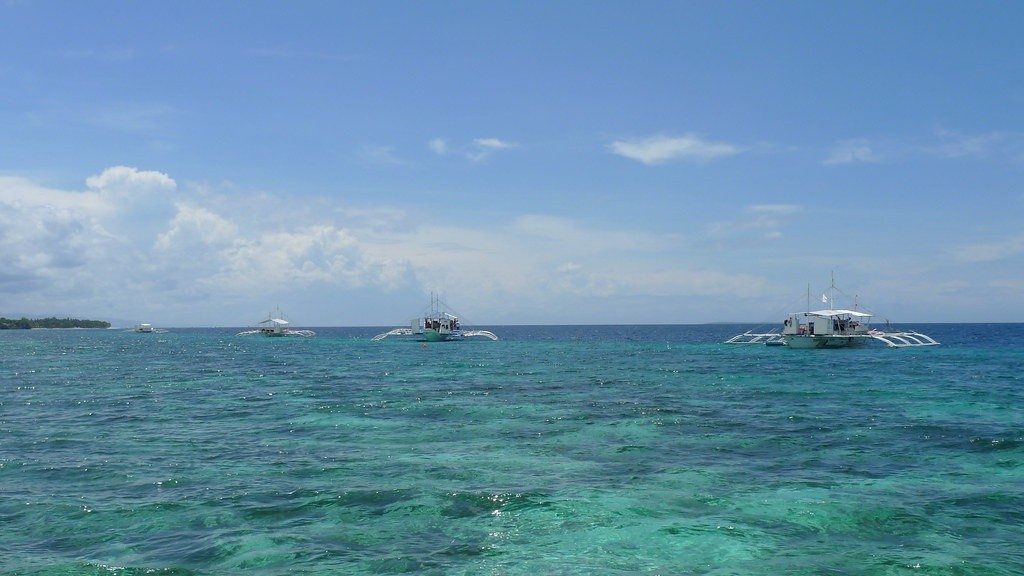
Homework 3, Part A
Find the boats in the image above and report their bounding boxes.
[122,322,169,333]
[371,291,498,342]
[724,269,941,348]
[235,304,316,337]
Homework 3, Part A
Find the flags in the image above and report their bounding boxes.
[823,293,827,302]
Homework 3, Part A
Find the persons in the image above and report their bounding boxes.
[800,327,805,335]
[427,322,431,328]
[451,320,460,330]
[433,321,440,332]
[789,317,792,327]
